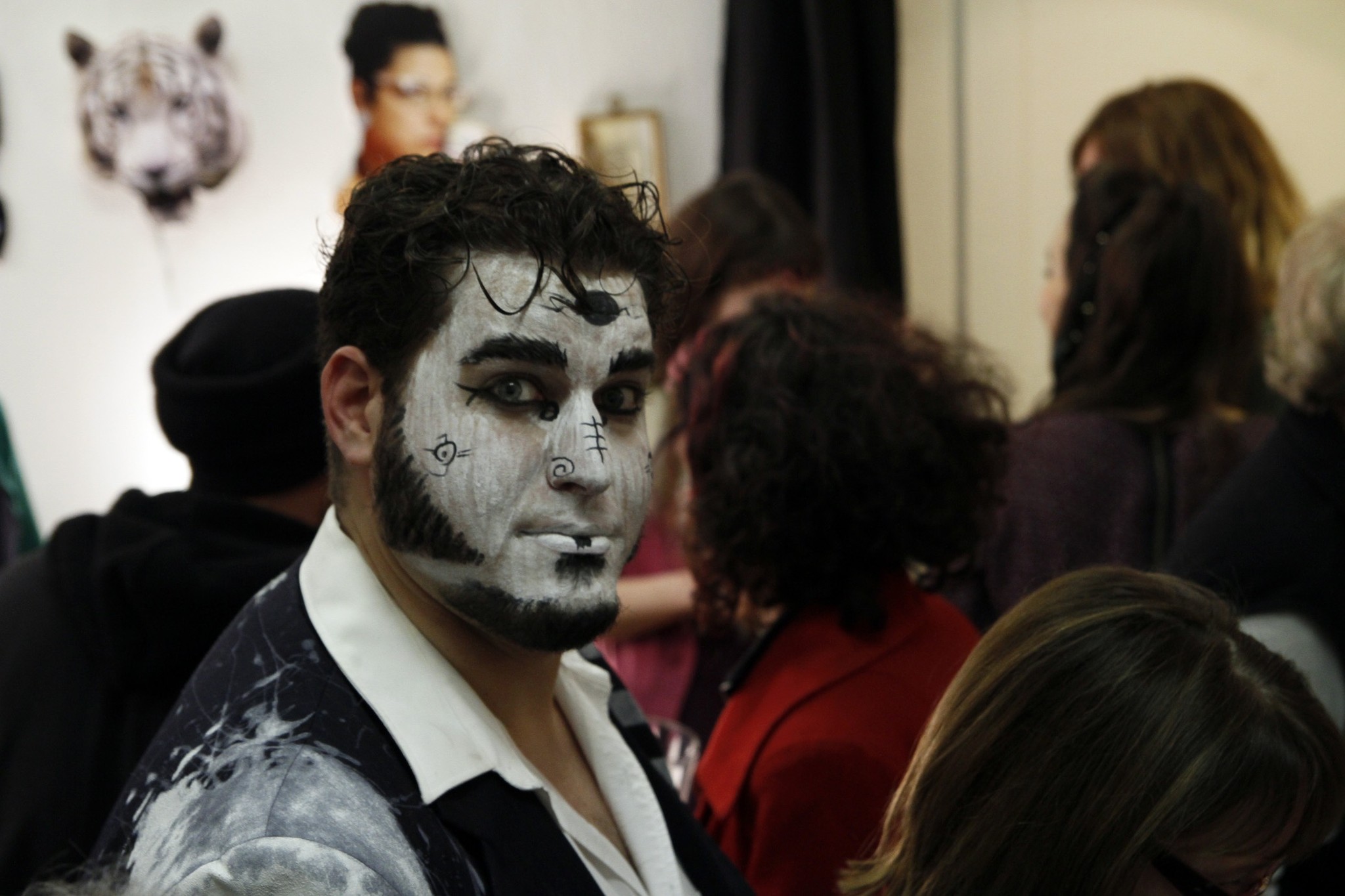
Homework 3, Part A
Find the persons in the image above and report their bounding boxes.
[662,286,1020,896]
[1065,76,1315,394]
[303,0,506,280]
[833,561,1345,896]
[964,161,1343,623]
[580,165,887,745]
[0,285,347,896]
[71,132,761,896]
